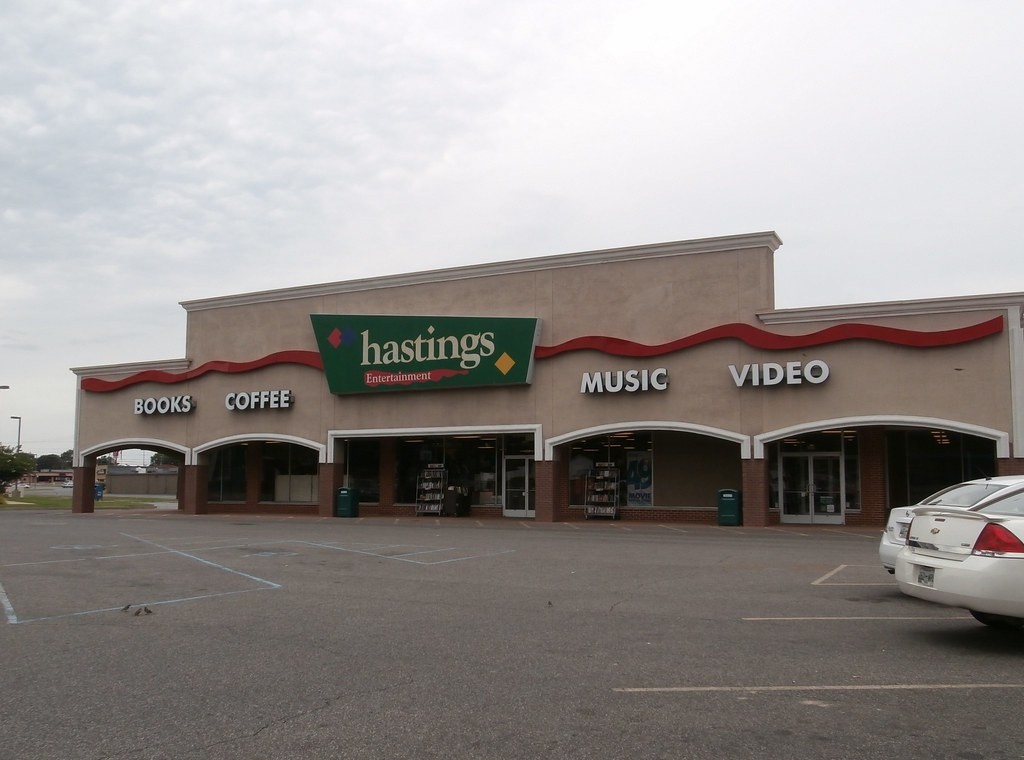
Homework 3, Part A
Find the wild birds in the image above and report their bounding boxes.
[121,604,132,612]
[547,600,553,607]
[134,606,152,616]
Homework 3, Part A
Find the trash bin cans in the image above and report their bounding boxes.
[94,484,103,501]
[718,487,741,526]
[335,486,359,517]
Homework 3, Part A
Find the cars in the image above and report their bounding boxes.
[61,481,73,488]
[879,474,1024,575]
[12,483,30,489]
[895,483,1024,632]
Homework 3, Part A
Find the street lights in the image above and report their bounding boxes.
[11,416,22,497]
